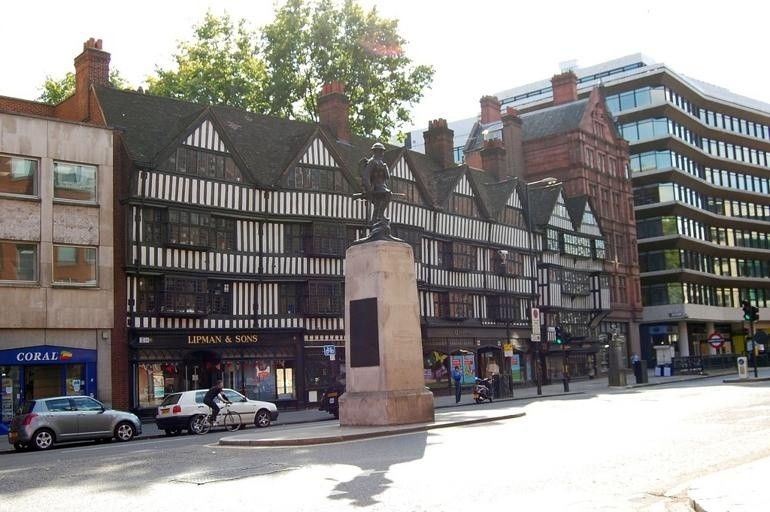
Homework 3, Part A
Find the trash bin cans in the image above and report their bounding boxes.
[492,374,514,400]
[634,360,648,384]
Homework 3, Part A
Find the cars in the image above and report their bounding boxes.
[154,388,278,437]
[7,395,142,451]
[317,372,346,420]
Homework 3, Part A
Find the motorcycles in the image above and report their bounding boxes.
[474,372,497,404]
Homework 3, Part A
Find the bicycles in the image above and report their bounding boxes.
[192,400,242,435]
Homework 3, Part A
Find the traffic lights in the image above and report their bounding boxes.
[742,299,753,321]
[753,305,759,320]
[564,331,571,344]
[555,325,563,345]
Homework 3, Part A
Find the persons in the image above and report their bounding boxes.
[361,139,395,227]
[452,365,464,405]
[203,379,230,425]
[630,351,641,377]
